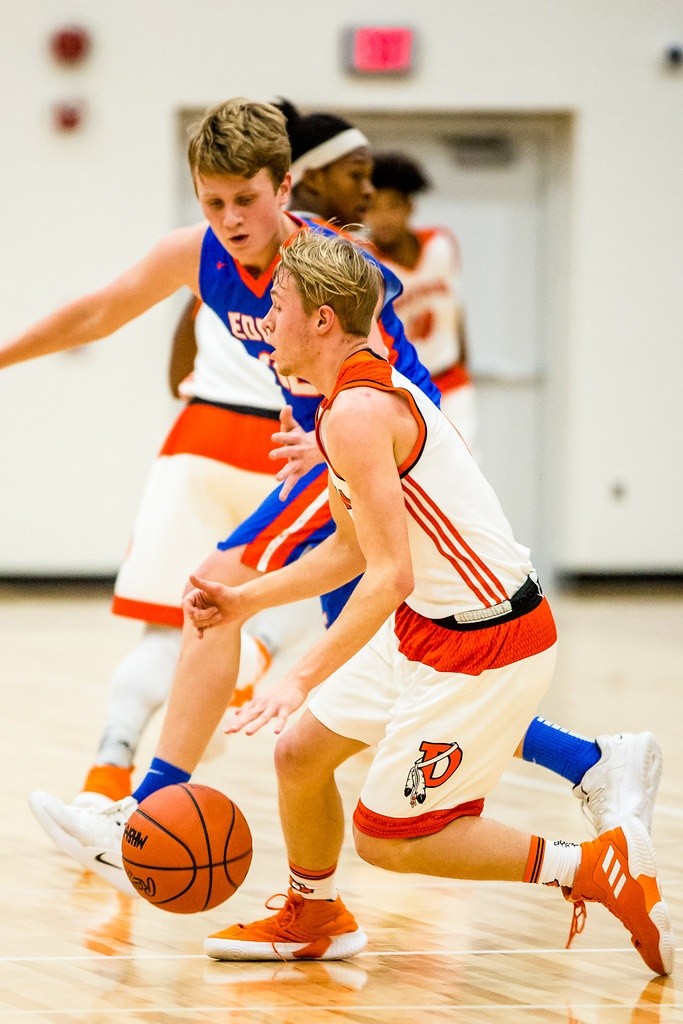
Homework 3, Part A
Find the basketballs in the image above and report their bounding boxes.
[121,782,252,914]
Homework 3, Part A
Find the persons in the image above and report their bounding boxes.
[187,229,671,980]
[337,154,475,442]
[61,101,390,849]
[0,101,664,883]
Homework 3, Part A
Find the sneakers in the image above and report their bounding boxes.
[561,816,674,977]
[25,789,144,898]
[572,731,663,841]
[204,887,369,961]
